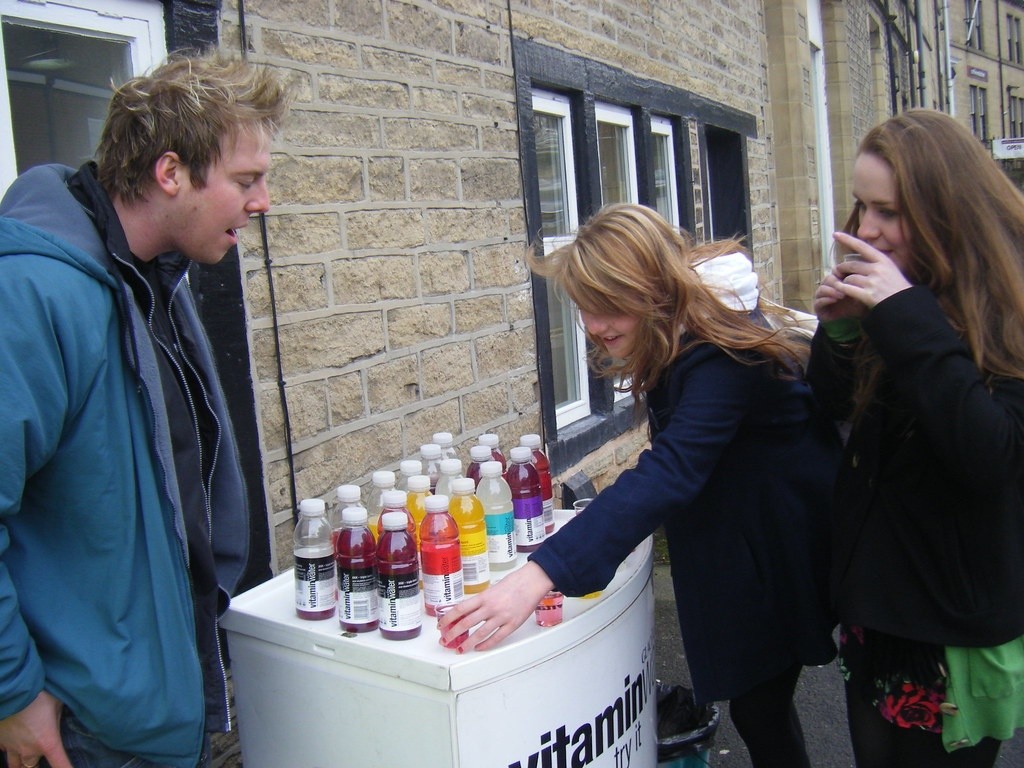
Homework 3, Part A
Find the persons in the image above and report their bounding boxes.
[0,43,300,768]
[804,109,1024,768]
[437,203,841,768]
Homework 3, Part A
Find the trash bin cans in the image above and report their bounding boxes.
[655,684,721,768]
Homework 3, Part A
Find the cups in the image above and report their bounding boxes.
[534,590,563,627]
[838,254,869,313]
[573,499,593,515]
[434,601,469,649]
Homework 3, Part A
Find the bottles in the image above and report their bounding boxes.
[377,512,421,640]
[334,507,381,632]
[467,434,507,495]
[377,491,418,547]
[435,459,465,499]
[405,475,433,590]
[332,486,364,603]
[397,460,422,492]
[419,432,457,496]
[292,498,336,620]
[519,433,555,533]
[449,478,491,596]
[419,495,465,616]
[366,471,396,546]
[477,461,518,571]
[506,447,547,553]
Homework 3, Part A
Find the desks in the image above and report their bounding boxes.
[219,509,659,768]
